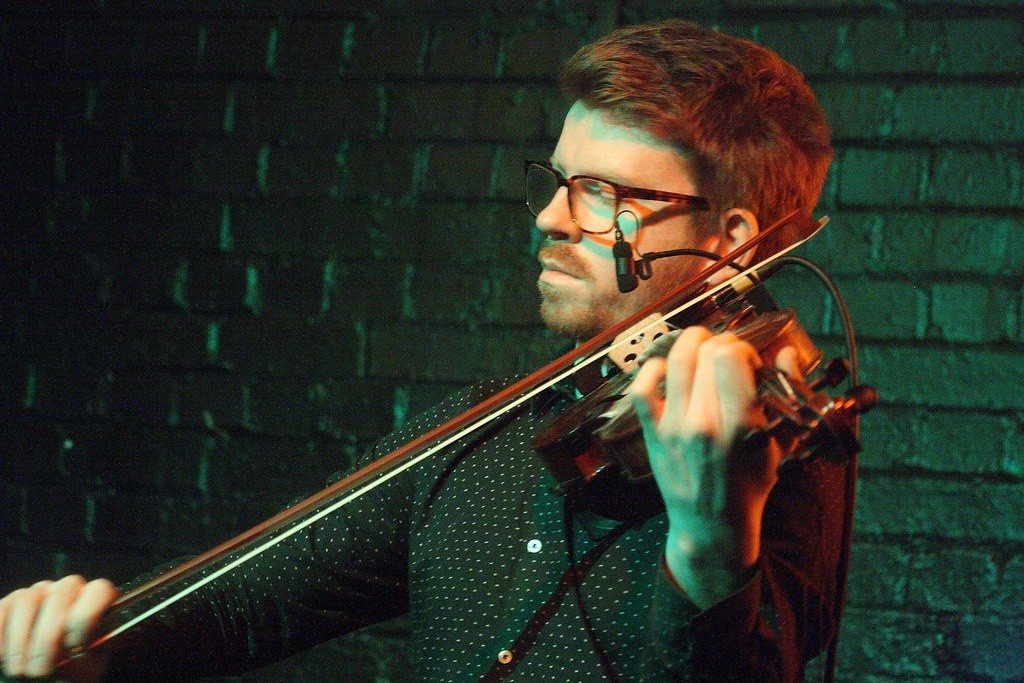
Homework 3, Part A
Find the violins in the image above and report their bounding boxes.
[528,294,876,497]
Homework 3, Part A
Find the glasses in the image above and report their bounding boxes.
[524,156,706,235]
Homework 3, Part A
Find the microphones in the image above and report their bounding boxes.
[613,239,760,294]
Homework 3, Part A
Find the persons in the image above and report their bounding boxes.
[0,21,854,683]
